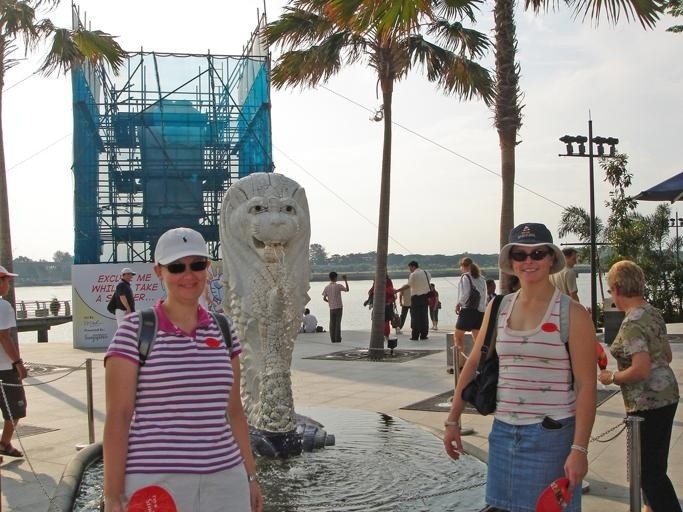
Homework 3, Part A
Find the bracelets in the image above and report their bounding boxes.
[14,359,22,364]
[611,371,617,385]
[570,444,588,455]
[445,422,458,426]
[249,474,260,481]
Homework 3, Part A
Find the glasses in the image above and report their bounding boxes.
[510,251,552,261]
[167,262,206,273]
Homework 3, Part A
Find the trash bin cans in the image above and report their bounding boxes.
[602,297,626,344]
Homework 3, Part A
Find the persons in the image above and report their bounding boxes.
[301,308,318,332]
[103,226,262,512]
[598,260,683,511]
[115,268,137,329]
[322,272,350,343]
[0,264,28,465]
[363,248,580,380]
[442,224,596,512]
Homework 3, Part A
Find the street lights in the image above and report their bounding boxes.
[558,121,621,329]
[669,209,683,274]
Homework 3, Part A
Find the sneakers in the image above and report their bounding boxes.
[396,326,400,334]
[410,336,428,340]
[446,366,463,374]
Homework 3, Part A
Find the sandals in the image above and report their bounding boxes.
[0,441,24,457]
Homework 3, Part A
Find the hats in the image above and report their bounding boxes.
[498,223,565,276]
[122,268,136,276]
[0,266,18,280]
[155,228,213,266]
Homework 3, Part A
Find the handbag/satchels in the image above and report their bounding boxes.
[438,301,441,309]
[391,312,401,327]
[469,288,480,312]
[317,326,323,332]
[427,291,437,305]
[462,350,499,415]
[107,295,116,314]
